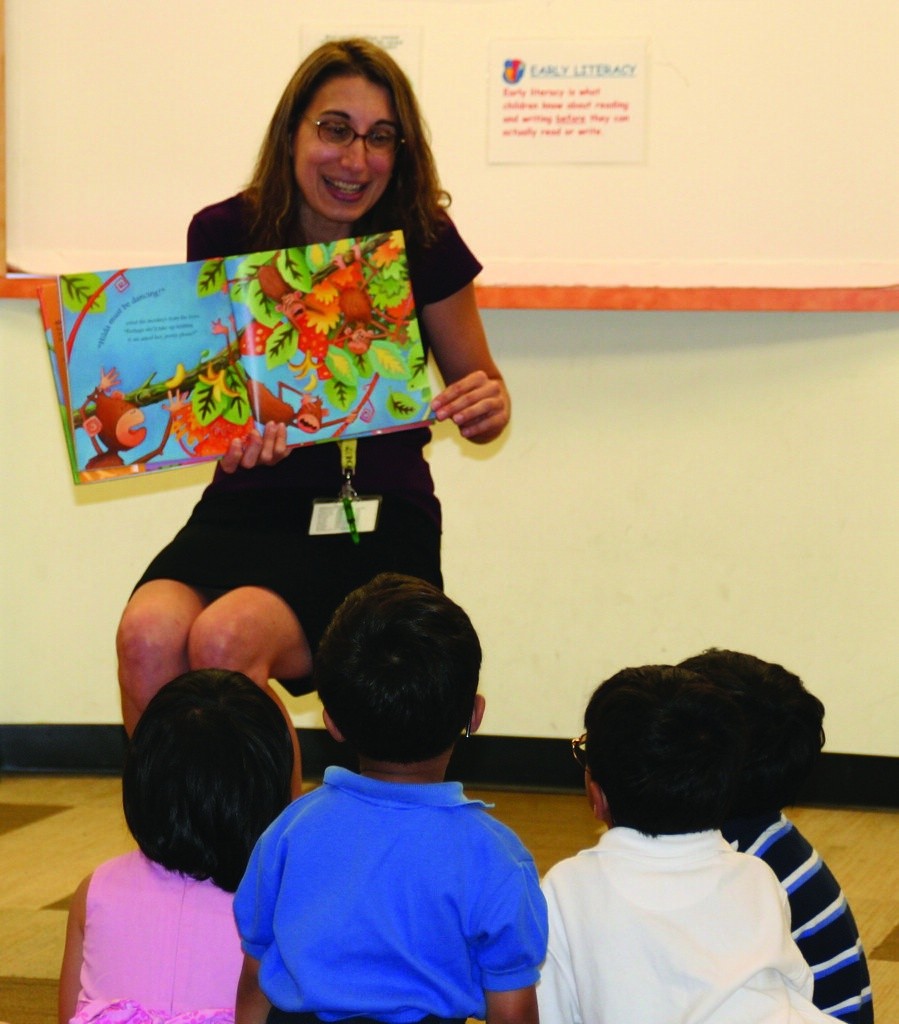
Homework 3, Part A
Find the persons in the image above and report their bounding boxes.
[58,666,294,1024]
[535,647,873,1024]
[116,37,511,802]
[234,573,548,1024]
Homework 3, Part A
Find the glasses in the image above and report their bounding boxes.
[304,114,405,157]
[571,733,593,778]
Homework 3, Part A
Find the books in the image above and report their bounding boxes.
[31,229,439,487]
